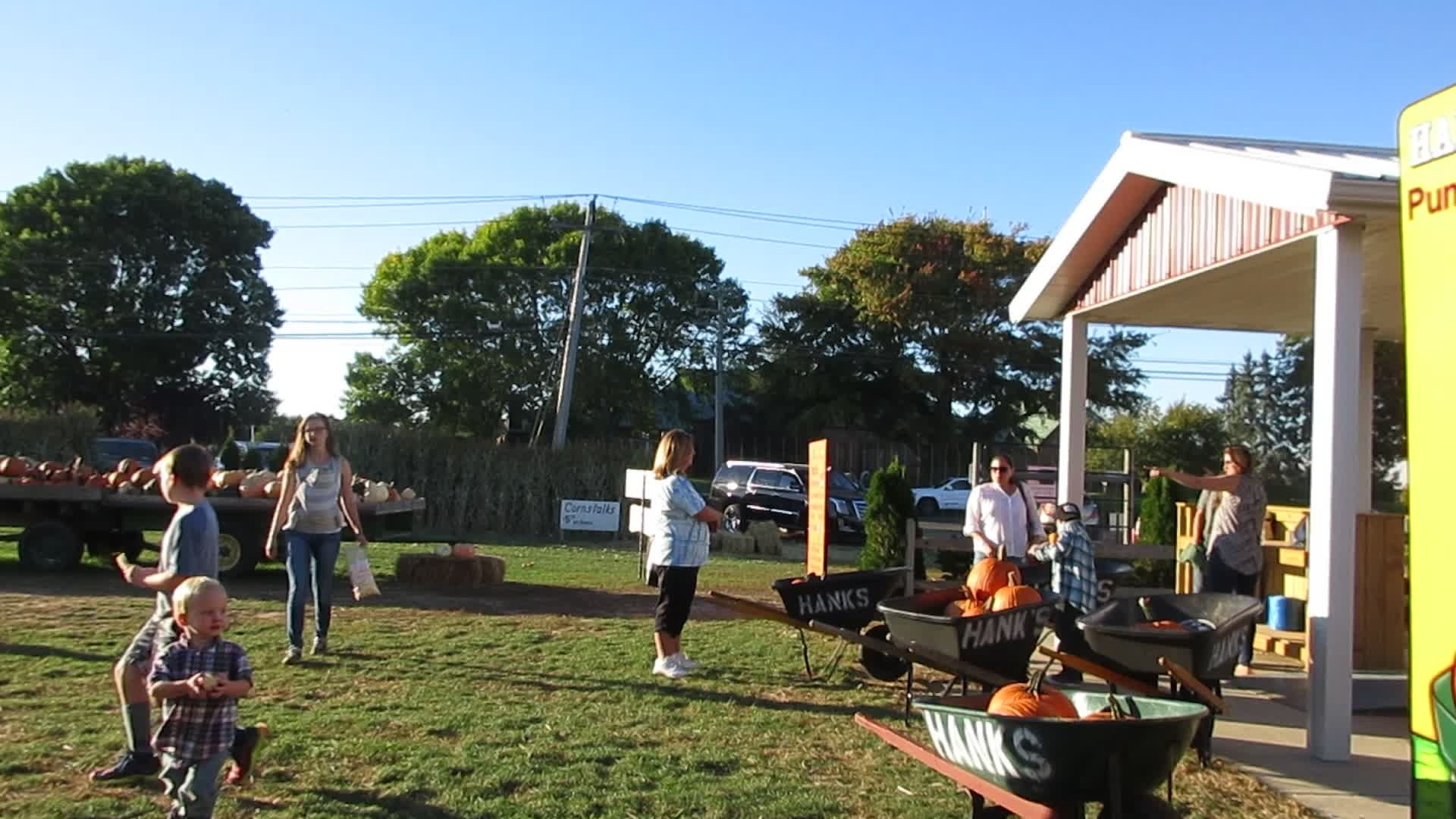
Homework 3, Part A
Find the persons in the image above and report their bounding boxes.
[147,575,253,819]
[265,412,367,662]
[92,444,268,786]
[1143,444,1268,674]
[652,429,719,678]
[964,454,1044,585]
[1028,501,1098,683]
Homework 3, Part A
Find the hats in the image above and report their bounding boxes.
[1055,503,1080,522]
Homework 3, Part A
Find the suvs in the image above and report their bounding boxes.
[707,457,871,545]
[1037,491,1100,540]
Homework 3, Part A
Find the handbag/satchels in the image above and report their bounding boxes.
[345,543,383,602]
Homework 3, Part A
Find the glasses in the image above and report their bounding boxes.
[304,427,328,434]
[990,466,1011,474]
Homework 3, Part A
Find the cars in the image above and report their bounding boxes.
[907,476,984,516]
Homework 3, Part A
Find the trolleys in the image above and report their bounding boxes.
[850,689,1210,819]
[1041,589,1265,754]
[709,566,1065,692]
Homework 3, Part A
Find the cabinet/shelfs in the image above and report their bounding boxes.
[1176,503,1409,670]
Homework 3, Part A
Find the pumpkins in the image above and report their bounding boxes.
[434,542,475,558]
[986,669,1141,719]
[966,544,1022,600]
[941,585,994,618]
[1130,597,1185,630]
[0,451,417,504]
[990,571,1044,611]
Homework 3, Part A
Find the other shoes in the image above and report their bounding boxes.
[310,634,326,656]
[1233,662,1250,677]
[1046,671,1082,687]
[282,645,301,668]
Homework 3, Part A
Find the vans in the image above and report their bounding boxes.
[85,436,159,472]
[212,441,289,472]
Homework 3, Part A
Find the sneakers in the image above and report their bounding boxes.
[672,653,700,668]
[652,656,691,680]
[228,721,268,785]
[87,746,161,785]
